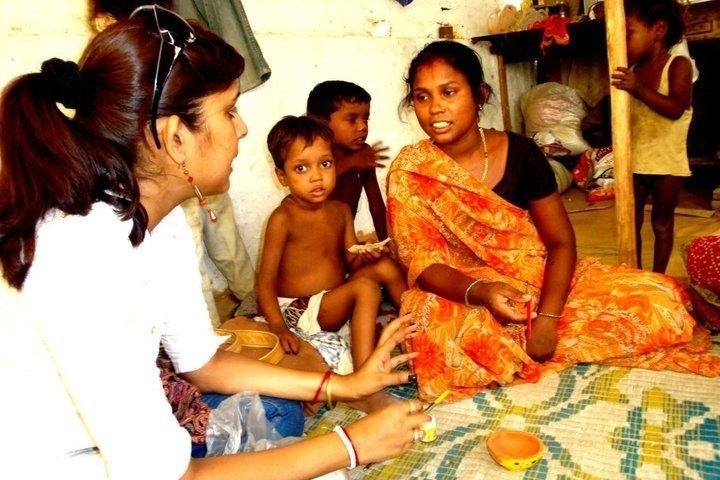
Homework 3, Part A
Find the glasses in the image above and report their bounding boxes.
[130,4,197,150]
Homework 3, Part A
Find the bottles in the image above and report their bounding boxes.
[418,415,438,444]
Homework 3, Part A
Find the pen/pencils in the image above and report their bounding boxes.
[424,388,449,414]
[526,300,532,338]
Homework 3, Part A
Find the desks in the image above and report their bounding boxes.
[471,18,610,136]
